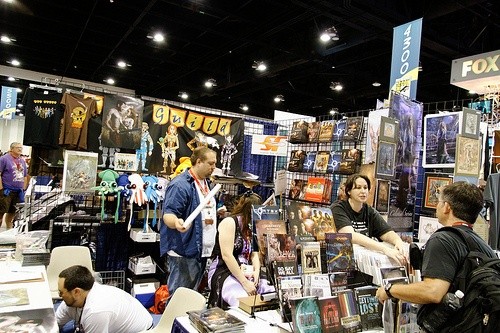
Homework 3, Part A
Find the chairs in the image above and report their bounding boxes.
[47,246,93,300]
[137,286,207,333]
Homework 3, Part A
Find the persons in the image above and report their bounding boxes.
[160,146,228,297]
[331,174,407,264]
[55,265,154,333]
[289,207,312,236]
[376,183,499,333]
[104,100,136,147]
[205,190,276,308]
[313,256,318,268]
[0,142,29,229]
[0,315,43,329]
[285,234,294,251]
[306,255,311,268]
[312,210,330,221]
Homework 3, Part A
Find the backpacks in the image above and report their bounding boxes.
[415,226,500,333]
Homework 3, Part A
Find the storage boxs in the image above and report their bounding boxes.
[130,228,156,243]
[154,231,160,241]
[133,276,160,309]
[138,209,163,219]
[128,259,157,275]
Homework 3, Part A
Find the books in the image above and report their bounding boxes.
[250,204,279,251]
[285,205,316,243]
[250,221,380,333]
[312,207,337,241]
[186,294,280,333]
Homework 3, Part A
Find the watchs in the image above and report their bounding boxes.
[385,282,394,299]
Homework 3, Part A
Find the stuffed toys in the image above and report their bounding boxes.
[92,157,192,231]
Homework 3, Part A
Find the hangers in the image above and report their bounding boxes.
[66,83,97,99]
[29,77,63,93]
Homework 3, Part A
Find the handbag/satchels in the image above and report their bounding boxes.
[150,286,168,315]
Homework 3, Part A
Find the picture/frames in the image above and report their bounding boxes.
[374,178,391,215]
[421,111,463,168]
[62,150,99,197]
[375,136,396,181]
[460,107,481,140]
[379,116,399,144]
[421,172,454,213]
[455,134,481,177]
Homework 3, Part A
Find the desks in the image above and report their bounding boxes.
[0,260,59,333]
[169,306,294,333]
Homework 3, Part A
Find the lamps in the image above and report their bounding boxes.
[329,81,343,92]
[204,77,217,90]
[146,28,154,39]
[239,103,249,112]
[178,91,189,101]
[251,59,268,73]
[328,108,340,116]
[319,25,339,43]
[273,94,285,104]
